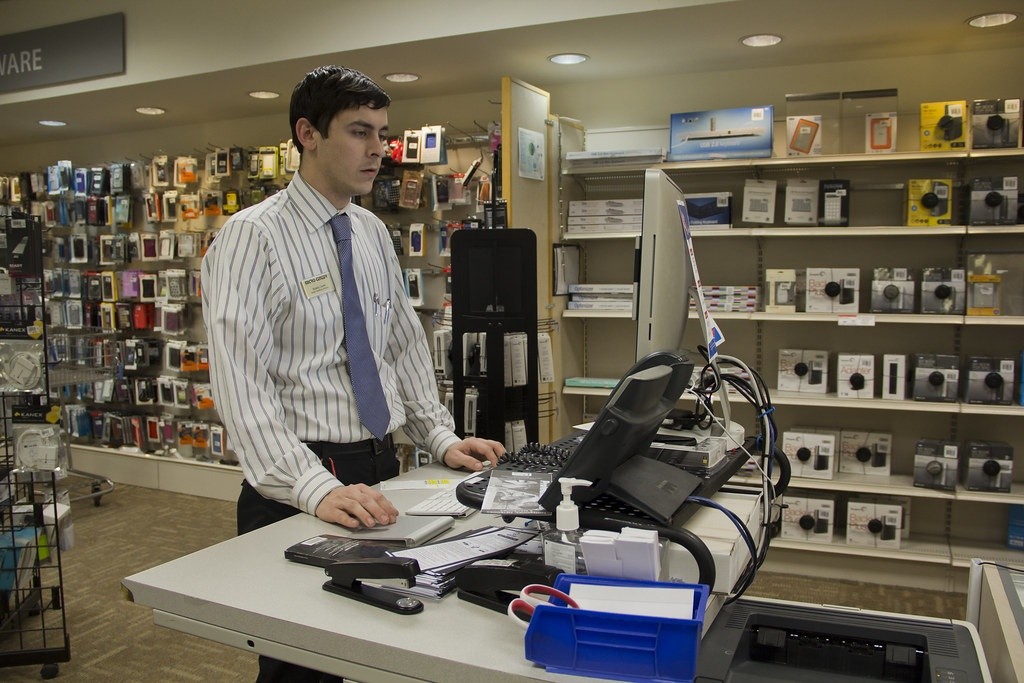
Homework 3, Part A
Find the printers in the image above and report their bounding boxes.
[692,593,992,683]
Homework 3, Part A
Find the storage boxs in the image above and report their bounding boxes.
[568,199,644,233]
[912,352,1015,405]
[781,487,911,549]
[445,388,478,437]
[914,437,1014,492]
[919,99,1021,151]
[408,223,427,256]
[667,105,773,161]
[903,177,1019,226]
[836,351,909,400]
[524,574,709,683]
[871,266,965,315]
[778,350,834,394]
[1007,504,1024,549]
[568,284,634,310]
[211,427,227,457]
[568,150,662,169]
[433,329,450,370]
[965,252,1024,316]
[684,179,850,231]
[763,267,860,314]
[782,425,892,480]
[565,378,620,389]
[689,286,761,314]
[785,89,899,157]
[57,489,69,506]
[563,148,968,595]
[669,492,761,594]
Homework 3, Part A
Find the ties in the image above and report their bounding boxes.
[329,215,391,441]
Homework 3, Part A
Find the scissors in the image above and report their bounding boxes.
[506,583,580,631]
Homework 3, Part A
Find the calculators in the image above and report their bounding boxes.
[823,189,844,223]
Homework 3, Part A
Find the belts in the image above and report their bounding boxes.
[301,435,393,458]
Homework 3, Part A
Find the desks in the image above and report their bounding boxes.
[123,461,729,682]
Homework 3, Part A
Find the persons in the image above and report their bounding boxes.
[200,66,508,683]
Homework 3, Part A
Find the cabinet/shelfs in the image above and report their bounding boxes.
[947,148,1024,568]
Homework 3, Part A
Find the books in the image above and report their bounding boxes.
[285,527,543,599]
[482,469,554,516]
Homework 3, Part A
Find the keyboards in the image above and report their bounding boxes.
[405,471,484,519]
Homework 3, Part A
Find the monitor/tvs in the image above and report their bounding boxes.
[635,169,730,440]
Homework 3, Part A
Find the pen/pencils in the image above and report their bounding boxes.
[371,288,380,320]
[384,298,392,322]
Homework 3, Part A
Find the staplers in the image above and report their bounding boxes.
[321,557,424,616]
[455,558,565,622]
